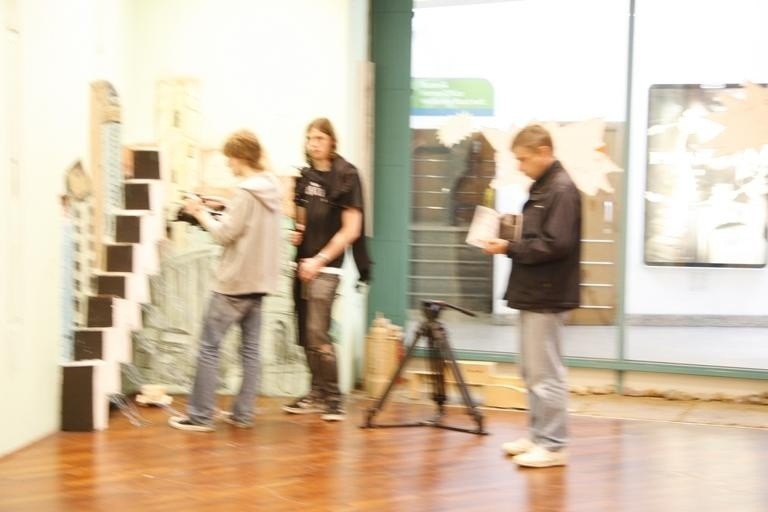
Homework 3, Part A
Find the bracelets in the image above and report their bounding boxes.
[318,251,332,263]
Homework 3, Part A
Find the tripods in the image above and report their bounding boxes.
[364,298,488,436]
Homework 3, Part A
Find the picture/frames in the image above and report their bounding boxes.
[642,81,767,268]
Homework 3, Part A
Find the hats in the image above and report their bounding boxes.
[223,130,266,170]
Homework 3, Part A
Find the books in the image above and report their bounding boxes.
[463,205,523,256]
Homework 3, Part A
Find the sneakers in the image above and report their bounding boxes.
[511,446,568,467]
[502,436,534,455]
[279,394,327,414]
[320,396,347,422]
[167,415,215,431]
[219,410,250,428]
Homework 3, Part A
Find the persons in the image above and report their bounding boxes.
[168,130,282,431]
[481,122,582,469]
[282,118,379,423]
